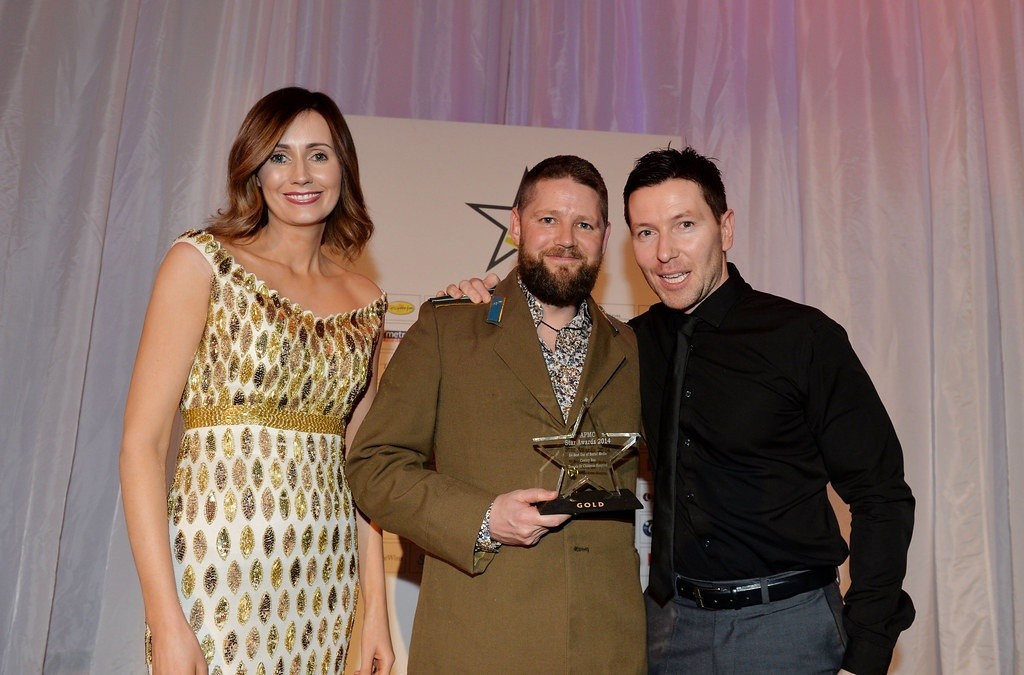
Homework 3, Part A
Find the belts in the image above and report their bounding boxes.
[676,566,838,610]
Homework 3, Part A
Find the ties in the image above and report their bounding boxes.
[648,316,695,609]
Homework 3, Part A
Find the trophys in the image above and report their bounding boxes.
[532,397,645,515]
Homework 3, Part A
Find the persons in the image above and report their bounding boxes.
[345,154,647,675]
[119,87,396,675]
[434,148,916,675]
